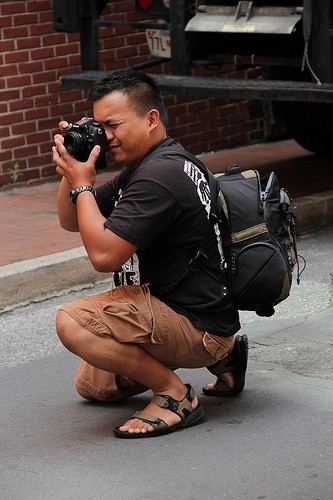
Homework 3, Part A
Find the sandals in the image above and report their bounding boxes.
[112,384,205,438]
[202,334,248,396]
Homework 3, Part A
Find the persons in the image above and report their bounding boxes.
[51,69,248,438]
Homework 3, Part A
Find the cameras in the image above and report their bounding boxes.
[63,116,107,169]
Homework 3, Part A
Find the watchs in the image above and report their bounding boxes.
[69,186,97,204]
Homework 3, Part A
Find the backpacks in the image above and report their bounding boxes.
[142,147,306,317]
